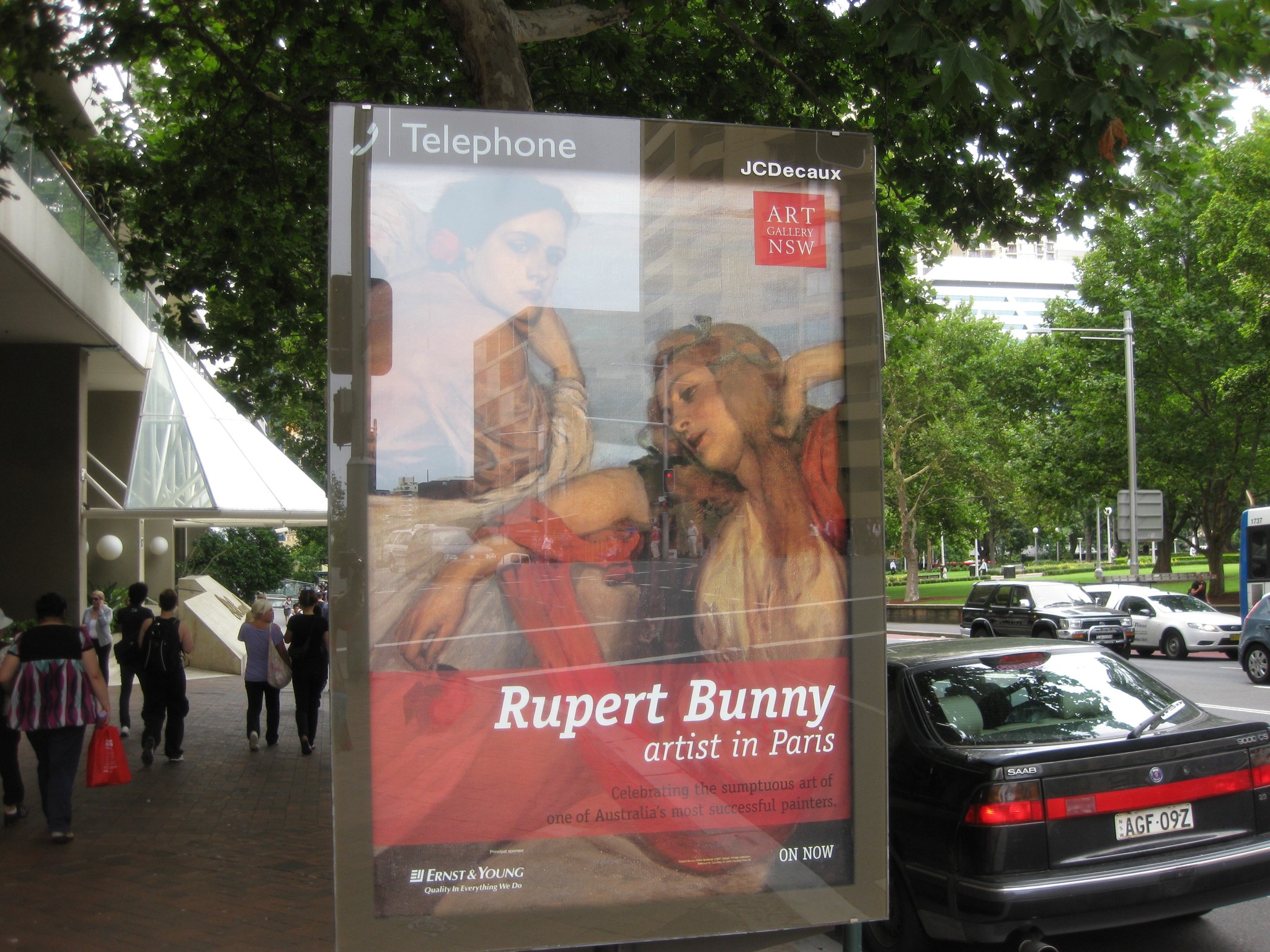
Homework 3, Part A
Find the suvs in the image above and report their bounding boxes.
[1237,592,1270,685]
[960,579,1136,660]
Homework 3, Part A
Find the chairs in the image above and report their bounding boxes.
[998,594,1009,605]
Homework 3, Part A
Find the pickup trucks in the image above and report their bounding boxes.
[1079,584,1243,661]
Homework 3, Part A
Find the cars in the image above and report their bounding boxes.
[865,635,1269,952]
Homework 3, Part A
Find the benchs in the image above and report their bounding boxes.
[1092,562,1117,567]
[918,575,940,580]
[1001,563,1026,575]
[942,684,1111,735]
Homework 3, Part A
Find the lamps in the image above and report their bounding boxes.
[148,536,168,556]
[95,534,123,560]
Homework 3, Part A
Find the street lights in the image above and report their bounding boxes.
[1032,527,1038,562]
[1077,538,1083,561]
[1103,506,1112,562]
[1055,527,1061,561]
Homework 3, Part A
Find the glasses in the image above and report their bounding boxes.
[91,598,101,602]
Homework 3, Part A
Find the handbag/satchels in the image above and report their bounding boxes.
[112,640,123,664]
[267,624,292,689]
[87,726,132,787]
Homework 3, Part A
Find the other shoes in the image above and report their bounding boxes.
[300,738,315,754]
[5,803,17,815]
[268,741,278,746]
[249,731,260,750]
[121,726,130,735]
[168,754,184,762]
[141,737,154,764]
[51,830,74,841]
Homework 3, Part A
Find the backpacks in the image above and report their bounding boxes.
[140,619,181,673]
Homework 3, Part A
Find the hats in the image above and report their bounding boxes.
[0,608,14,629]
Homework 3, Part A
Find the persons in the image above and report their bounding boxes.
[0,608,33,824]
[238,599,293,750]
[1189,575,1212,608]
[138,588,193,763]
[82,591,113,688]
[289,604,303,617]
[283,589,329,755]
[282,597,292,625]
[1142,543,1150,555]
[978,559,988,575]
[0,593,112,839]
[942,566,947,580]
[940,566,944,579]
[368,168,851,914]
[1126,549,1130,566]
[1110,547,1117,564]
[316,580,328,618]
[890,559,896,575]
[110,582,156,735]
